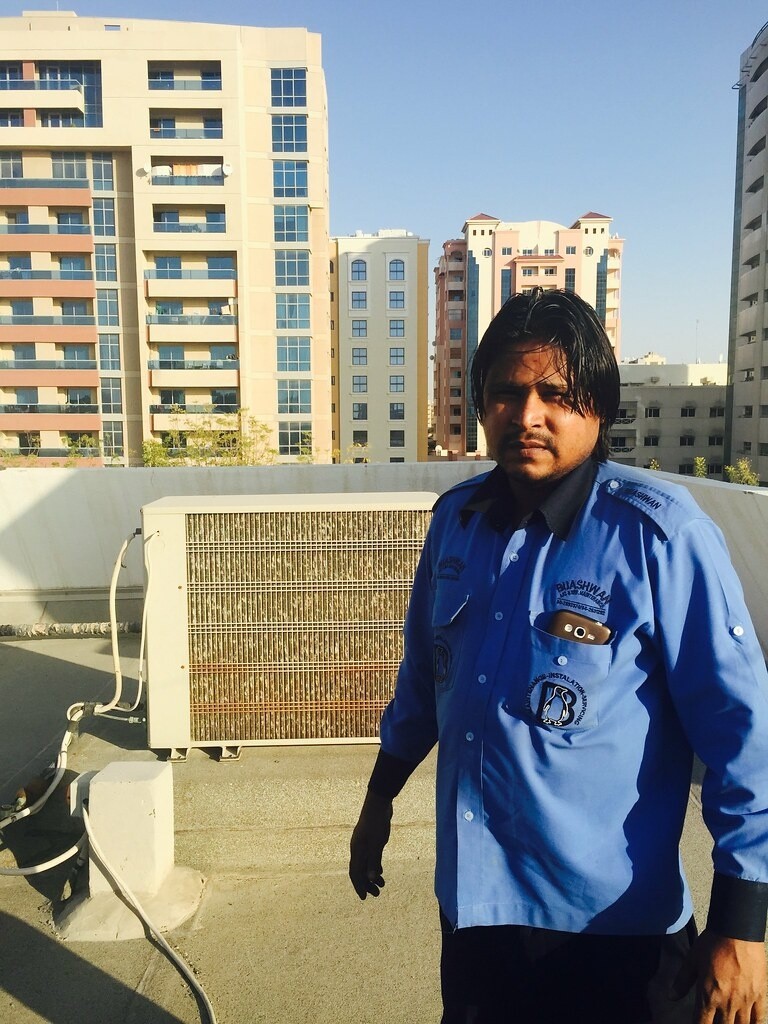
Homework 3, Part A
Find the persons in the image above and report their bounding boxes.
[348,283,768,1024]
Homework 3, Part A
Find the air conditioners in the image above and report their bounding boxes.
[137,492,441,749]
